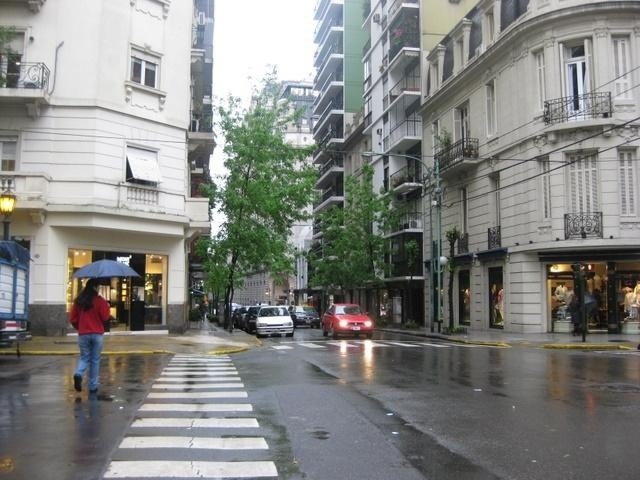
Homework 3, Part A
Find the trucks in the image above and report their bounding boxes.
[0,242,31,351]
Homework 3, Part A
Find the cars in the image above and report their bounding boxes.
[213,301,320,339]
[322,302,374,339]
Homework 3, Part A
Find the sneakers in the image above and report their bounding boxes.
[89,388,97,393]
[74,373,81,391]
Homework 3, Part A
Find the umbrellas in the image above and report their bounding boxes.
[67,259,143,303]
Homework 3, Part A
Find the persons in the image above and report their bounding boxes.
[67,279,112,395]
[461,275,640,334]
[199,300,209,322]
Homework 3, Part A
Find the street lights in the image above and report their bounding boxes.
[0,178,17,245]
[362,148,447,333]
